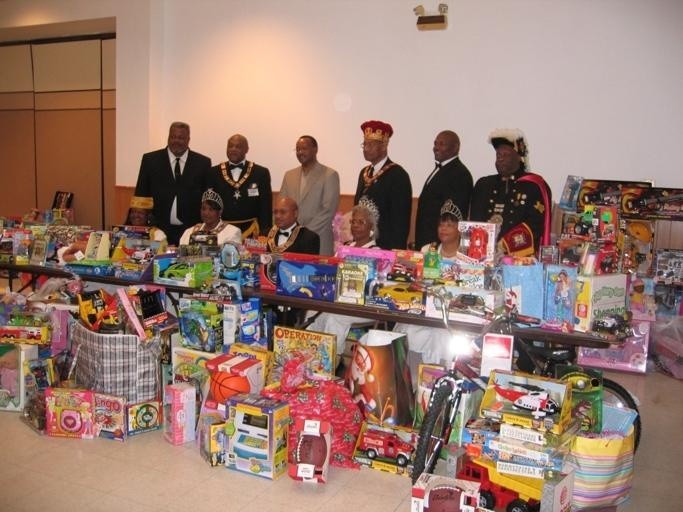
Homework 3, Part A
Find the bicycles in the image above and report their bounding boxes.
[410,286,641,487]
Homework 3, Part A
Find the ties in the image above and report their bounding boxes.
[175,158,182,185]
[368,167,374,176]
[301,169,309,194]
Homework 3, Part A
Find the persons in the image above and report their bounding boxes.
[334,196,380,257]
[124,196,154,226]
[420,199,465,262]
[211,133,274,239]
[279,135,340,256]
[353,120,413,251]
[180,187,243,247]
[0,344,20,409]
[414,129,474,251]
[469,128,553,257]
[133,122,211,247]
[263,196,321,255]
[629,278,648,313]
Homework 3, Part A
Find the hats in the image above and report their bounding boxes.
[488,128,529,170]
[362,120,391,142]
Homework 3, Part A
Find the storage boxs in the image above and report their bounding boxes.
[653,217,683,251]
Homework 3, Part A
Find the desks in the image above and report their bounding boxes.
[2,264,204,311]
[241,284,609,374]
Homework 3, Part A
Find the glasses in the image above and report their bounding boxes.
[295,146,311,151]
[361,142,381,148]
[349,219,369,224]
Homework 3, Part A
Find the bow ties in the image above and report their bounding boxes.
[228,163,243,169]
[277,231,289,237]
[503,175,514,180]
[435,163,441,167]
